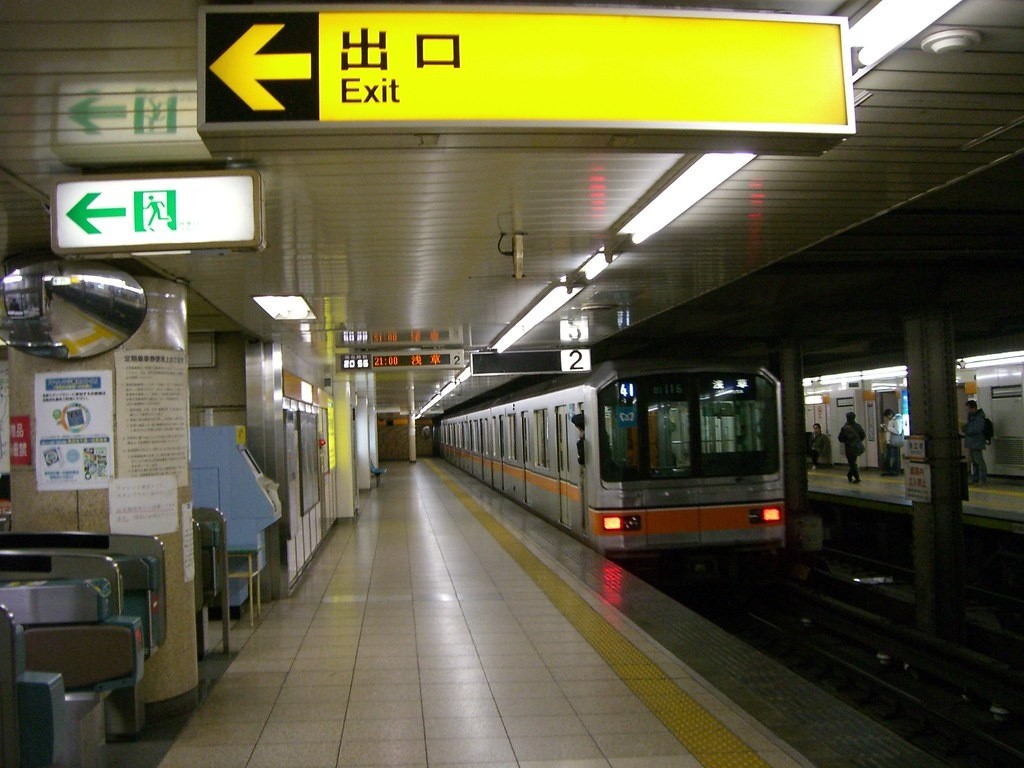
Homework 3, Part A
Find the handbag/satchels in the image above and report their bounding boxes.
[856,442,865,457]
[890,429,905,447]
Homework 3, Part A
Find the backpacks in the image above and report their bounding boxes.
[983,419,994,445]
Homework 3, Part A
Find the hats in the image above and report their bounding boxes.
[571,414,584,428]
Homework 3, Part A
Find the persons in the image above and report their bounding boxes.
[809,422,829,471]
[838,412,866,484]
[8,298,20,311]
[42,282,54,309]
[881,409,905,475]
[962,400,987,485]
[571,414,629,482]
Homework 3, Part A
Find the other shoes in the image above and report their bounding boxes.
[811,465,817,470]
[890,472,898,476]
[847,473,852,482]
[881,471,888,475]
[852,479,861,483]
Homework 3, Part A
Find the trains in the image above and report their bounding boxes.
[435,355,788,561]
